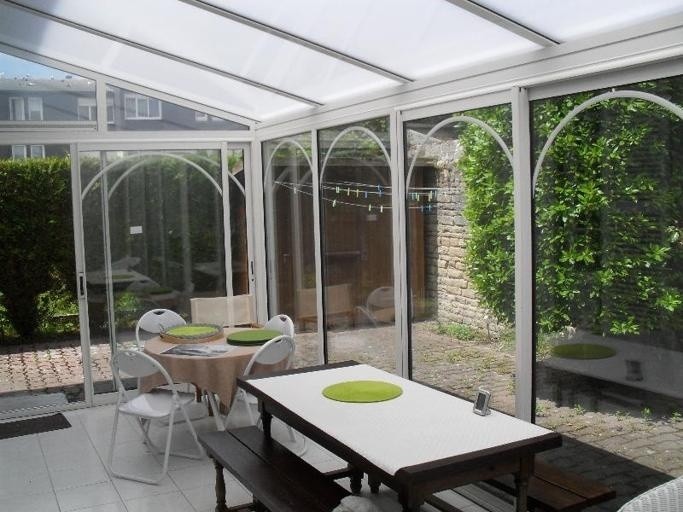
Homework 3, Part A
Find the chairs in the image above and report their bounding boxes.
[224,335,310,456]
[366,284,396,327]
[135,308,210,426]
[107,349,205,485]
[264,313,295,340]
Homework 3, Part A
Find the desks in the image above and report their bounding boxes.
[542,329,682,409]
[139,327,296,445]
[89,289,180,329]
[236,360,562,512]
[86,267,152,289]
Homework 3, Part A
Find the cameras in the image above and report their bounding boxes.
[473,388,493,417]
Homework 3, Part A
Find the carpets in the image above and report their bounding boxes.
[0,413,71,442]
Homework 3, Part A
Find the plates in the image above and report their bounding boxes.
[157,320,225,339]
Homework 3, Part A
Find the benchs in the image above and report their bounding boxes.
[197,424,355,511]
[368,457,618,512]
